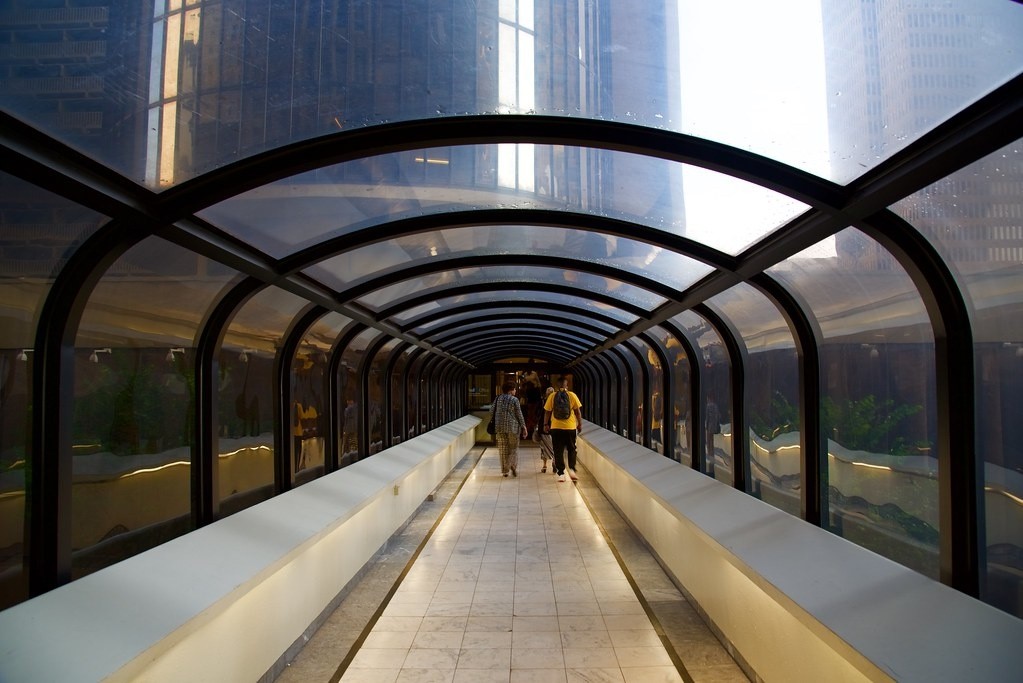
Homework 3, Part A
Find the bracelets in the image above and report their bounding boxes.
[545,424,549,426]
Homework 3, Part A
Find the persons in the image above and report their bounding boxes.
[519,359,543,439]
[490,381,528,477]
[543,377,582,482]
[295,393,381,474]
[639,381,722,461]
[534,387,557,473]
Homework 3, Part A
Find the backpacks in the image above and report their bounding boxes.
[553,389,570,420]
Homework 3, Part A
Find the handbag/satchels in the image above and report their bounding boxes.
[531,416,542,443]
[487,394,500,435]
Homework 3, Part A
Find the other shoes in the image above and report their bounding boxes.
[557,474,566,481]
[569,469,578,482]
[541,466,547,473]
[511,466,517,477]
[503,473,508,477]
